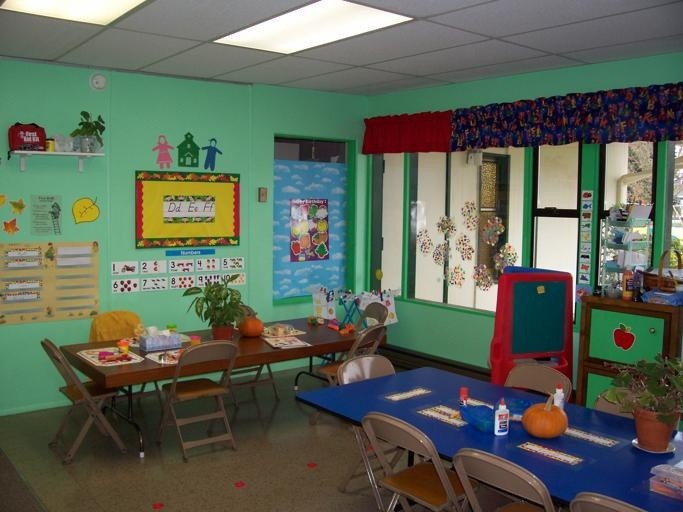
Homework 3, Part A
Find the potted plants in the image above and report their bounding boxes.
[180,273,244,342]
[68,110,107,151]
[605,352,682,450]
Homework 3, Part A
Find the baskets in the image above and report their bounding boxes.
[644,249,683,293]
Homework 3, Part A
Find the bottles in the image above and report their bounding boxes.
[623,265,634,299]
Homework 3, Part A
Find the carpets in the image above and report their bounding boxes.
[0,369,452,512]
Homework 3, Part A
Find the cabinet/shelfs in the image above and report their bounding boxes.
[600,216,654,296]
[576,294,682,409]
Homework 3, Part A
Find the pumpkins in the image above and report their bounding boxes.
[238,314,264,337]
[299,234,311,250]
[317,217,327,232]
[522,393,568,439]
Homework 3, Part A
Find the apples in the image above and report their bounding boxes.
[613,323,634,349]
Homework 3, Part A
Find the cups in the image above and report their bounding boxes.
[190,335,201,345]
[118,339,128,354]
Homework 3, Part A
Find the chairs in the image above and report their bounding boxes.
[216,303,281,410]
[339,299,388,360]
[307,322,386,426]
[38,337,129,464]
[154,341,239,463]
[87,310,167,428]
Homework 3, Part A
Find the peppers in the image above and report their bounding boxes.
[291,242,300,255]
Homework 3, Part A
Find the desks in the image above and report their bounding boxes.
[293,365,683,511]
[57,315,387,460]
